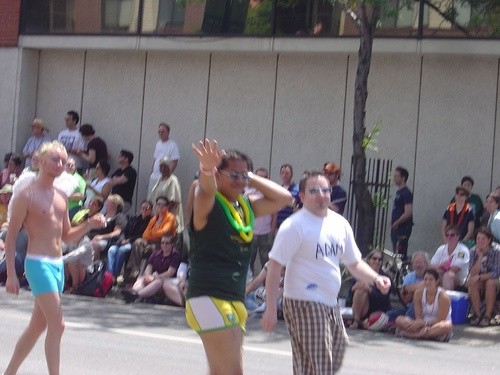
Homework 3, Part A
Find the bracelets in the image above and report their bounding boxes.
[200,164,218,176]
[425,320,429,326]
[479,275,481,280]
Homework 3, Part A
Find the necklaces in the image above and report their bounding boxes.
[217,191,253,242]
[215,192,254,232]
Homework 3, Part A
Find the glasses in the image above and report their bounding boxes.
[161,242,170,245]
[310,188,332,196]
[446,233,454,237]
[158,131,164,133]
[221,171,248,184]
[141,206,150,211]
[372,257,383,261]
[456,192,465,196]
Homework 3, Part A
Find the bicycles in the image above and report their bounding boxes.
[381,235,414,309]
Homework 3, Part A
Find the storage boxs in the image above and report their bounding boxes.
[447,289,469,324]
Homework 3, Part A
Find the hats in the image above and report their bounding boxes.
[324,162,339,174]
[158,157,178,174]
[28,119,49,132]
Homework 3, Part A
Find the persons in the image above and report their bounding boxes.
[185,138,293,375]
[391,167,413,263]
[326,162,347,215]
[344,249,394,329]
[396,268,453,342]
[246,165,310,319]
[431,226,470,291]
[401,251,430,319]
[262,169,391,375]
[466,228,500,325]
[5,142,106,375]
[441,176,500,320]
[0,111,190,307]
